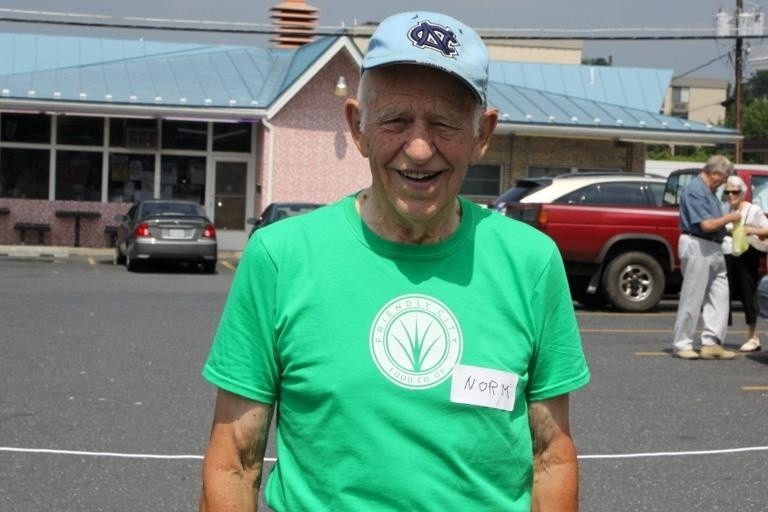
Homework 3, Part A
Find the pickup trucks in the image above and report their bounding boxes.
[506,167,768,312]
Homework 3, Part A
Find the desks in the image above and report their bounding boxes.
[55,207,101,246]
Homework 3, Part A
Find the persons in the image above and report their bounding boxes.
[717,173,768,353]
[193,7,591,512]
[670,154,742,360]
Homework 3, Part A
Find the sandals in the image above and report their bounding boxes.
[738,336,763,353]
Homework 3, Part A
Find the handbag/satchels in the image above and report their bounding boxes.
[746,234,768,254]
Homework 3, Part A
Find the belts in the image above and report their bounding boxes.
[682,231,723,245]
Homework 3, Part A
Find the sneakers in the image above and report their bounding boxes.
[672,349,700,360]
[698,344,736,361]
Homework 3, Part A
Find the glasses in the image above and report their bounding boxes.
[723,190,741,195]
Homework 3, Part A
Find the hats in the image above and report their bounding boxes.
[359,11,490,106]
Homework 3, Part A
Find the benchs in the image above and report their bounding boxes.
[11,219,52,243]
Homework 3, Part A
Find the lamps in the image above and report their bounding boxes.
[334,74,348,88]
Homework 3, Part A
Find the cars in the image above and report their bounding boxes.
[112,199,217,273]
[246,202,331,241]
[489,170,669,216]
